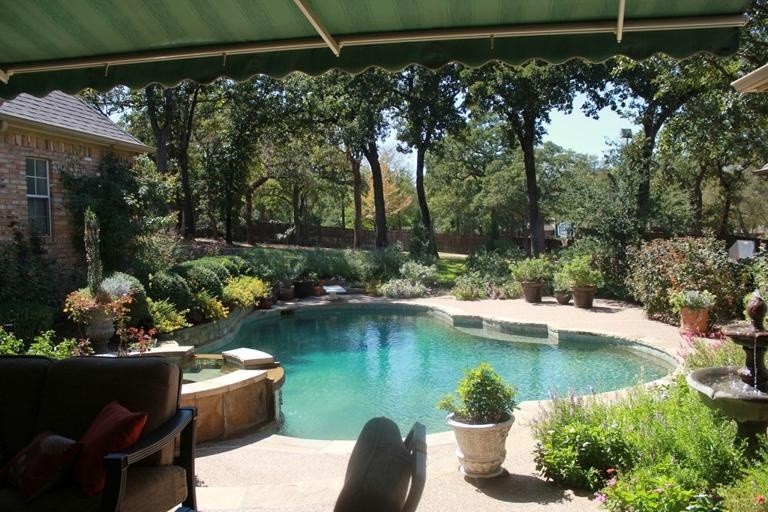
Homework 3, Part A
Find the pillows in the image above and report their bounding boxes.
[1,398,148,502]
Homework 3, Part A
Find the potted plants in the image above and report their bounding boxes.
[436,360,523,482]
[666,288,718,337]
[250,244,339,308]
[508,252,607,308]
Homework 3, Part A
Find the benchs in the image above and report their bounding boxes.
[0,352,198,511]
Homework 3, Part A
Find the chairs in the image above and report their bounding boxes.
[330,415,429,512]
